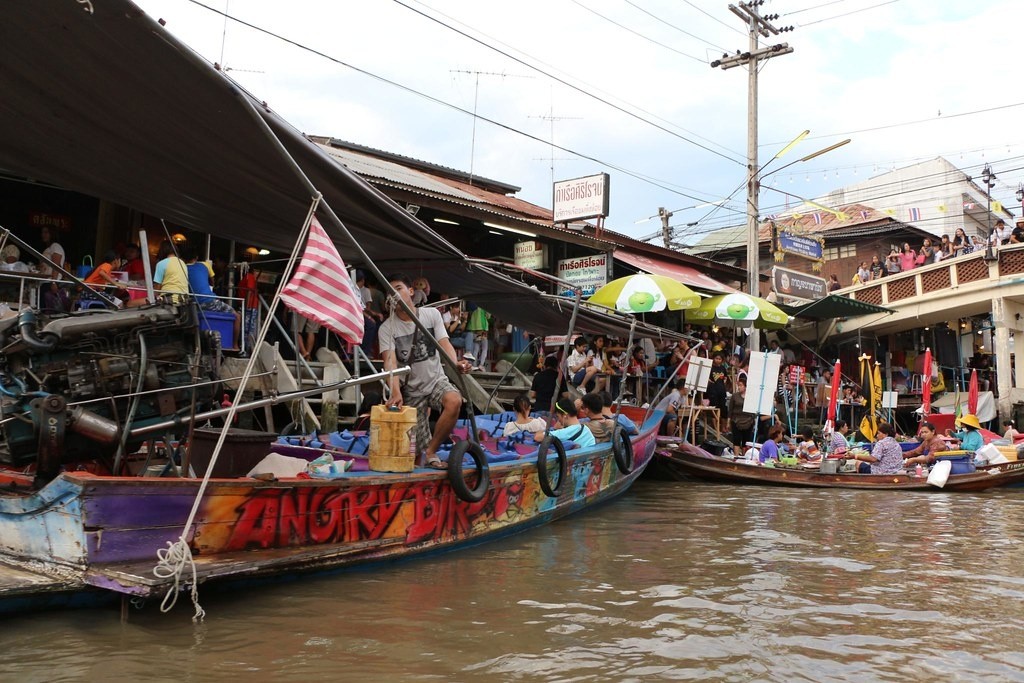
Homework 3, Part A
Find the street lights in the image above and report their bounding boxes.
[1016,180,1024,219]
[981,162,997,260]
[745,128,853,352]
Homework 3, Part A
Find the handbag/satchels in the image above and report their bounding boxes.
[913,252,927,265]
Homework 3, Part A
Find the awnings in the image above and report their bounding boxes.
[792,294,898,327]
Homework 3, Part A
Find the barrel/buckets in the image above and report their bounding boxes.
[701,439,729,456]
[142,465,182,477]
[198,311,236,349]
[369,404,417,472]
[933,450,976,474]
[820,458,841,473]
[76,266,94,278]
[864,442,929,456]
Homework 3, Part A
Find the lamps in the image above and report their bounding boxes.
[960,318,967,330]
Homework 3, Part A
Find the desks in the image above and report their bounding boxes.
[190,428,280,479]
[677,406,720,446]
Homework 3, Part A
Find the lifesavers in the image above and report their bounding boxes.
[613,426,634,476]
[448,440,490,503]
[537,435,567,498]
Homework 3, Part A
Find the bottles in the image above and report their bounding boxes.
[731,450,734,455]
[913,384,916,394]
[955,382,959,392]
[916,464,922,476]
[782,458,797,465]
[918,383,922,394]
[789,436,796,454]
[849,395,853,403]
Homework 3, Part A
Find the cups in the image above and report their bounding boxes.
[805,372,810,381]
[989,367,994,371]
[631,366,636,375]
[703,399,710,407]
[894,388,899,394]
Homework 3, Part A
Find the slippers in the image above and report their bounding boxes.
[423,458,448,470]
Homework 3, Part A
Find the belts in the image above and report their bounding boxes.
[467,329,476,332]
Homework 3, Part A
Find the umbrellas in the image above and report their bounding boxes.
[968,368,978,415]
[923,347,933,413]
[823,359,841,433]
[584,270,702,404]
[685,293,788,360]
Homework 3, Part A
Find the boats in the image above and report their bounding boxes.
[0,0,714,606]
[644,404,1024,493]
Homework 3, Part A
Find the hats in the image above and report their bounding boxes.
[1015,216,1024,223]
[955,414,982,430]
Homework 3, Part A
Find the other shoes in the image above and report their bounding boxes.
[478,365,486,372]
[619,390,633,395]
[470,365,479,371]
[615,399,629,404]
[666,443,679,448]
[463,352,475,361]
[576,385,587,396]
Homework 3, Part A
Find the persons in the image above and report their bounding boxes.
[577,391,639,435]
[1002,419,1018,440]
[378,272,471,469]
[81,244,144,305]
[290,311,322,361]
[581,394,615,443]
[702,328,856,432]
[851,216,1024,285]
[547,335,660,395]
[829,274,840,292]
[757,414,986,474]
[153,239,258,352]
[535,398,596,448]
[531,356,569,412]
[354,268,508,372]
[39,223,65,309]
[504,395,546,436]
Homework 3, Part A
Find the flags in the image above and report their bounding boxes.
[768,201,1002,221]
[279,215,365,345]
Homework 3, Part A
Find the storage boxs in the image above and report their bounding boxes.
[198,310,236,349]
